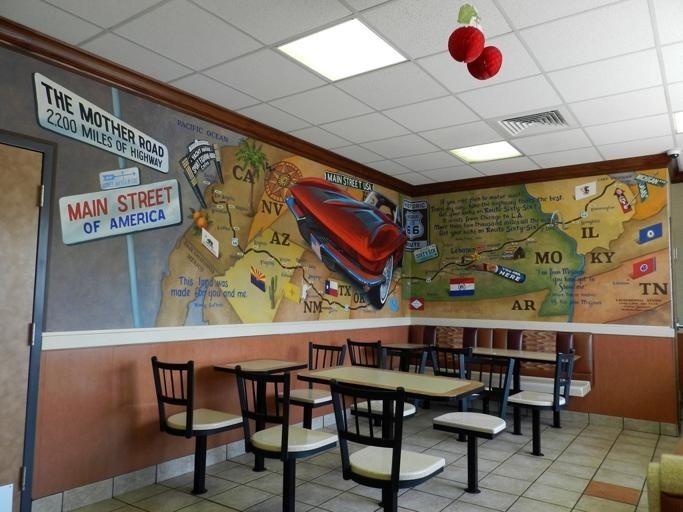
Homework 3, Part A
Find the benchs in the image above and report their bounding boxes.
[396,323,595,429]
